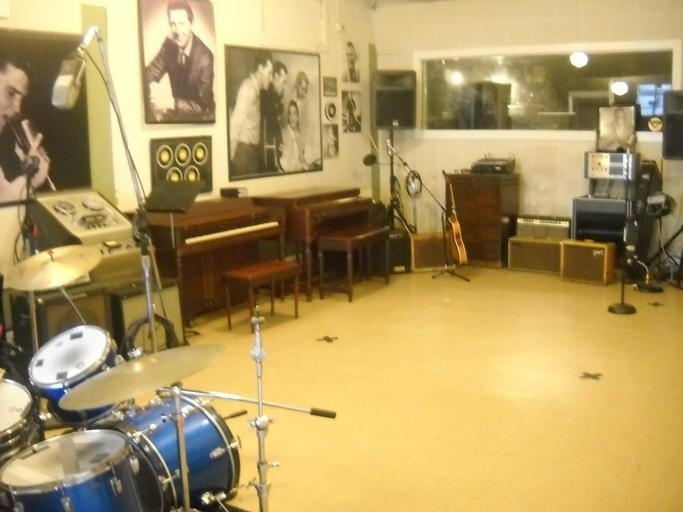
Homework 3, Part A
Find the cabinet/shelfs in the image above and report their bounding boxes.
[447,174,521,270]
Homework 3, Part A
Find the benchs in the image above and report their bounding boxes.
[223,260,300,334]
[318,222,391,303]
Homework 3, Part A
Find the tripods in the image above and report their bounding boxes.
[164,212,199,335]
[380,130,416,236]
[396,154,470,282]
[84,48,157,354]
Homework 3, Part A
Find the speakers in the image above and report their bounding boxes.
[663,90,683,159]
[371,69,416,129]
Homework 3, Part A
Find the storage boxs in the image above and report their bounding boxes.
[409,233,457,271]
[560,241,616,286]
[507,235,560,276]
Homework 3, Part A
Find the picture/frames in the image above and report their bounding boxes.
[224,43,325,182]
[321,124,339,158]
[321,78,338,97]
[342,35,361,83]
[596,103,637,152]
[342,90,364,133]
[0,28,93,209]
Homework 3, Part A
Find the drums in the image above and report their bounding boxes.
[0,377,45,465]
[110,385,243,512]
[28,323,129,428]
[1,428,144,512]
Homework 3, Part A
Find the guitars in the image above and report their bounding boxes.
[440,168,470,271]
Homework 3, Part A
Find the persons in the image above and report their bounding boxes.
[0,27,49,201]
[232,54,274,177]
[258,62,287,172]
[292,74,313,107]
[280,101,311,170]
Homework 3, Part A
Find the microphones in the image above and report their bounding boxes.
[12,118,56,192]
[52,59,86,108]
[626,133,637,149]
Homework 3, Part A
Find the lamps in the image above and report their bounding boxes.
[448,69,465,84]
[610,81,627,97]
[569,51,591,71]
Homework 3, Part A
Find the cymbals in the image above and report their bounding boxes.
[7,244,102,292]
[58,343,224,411]
